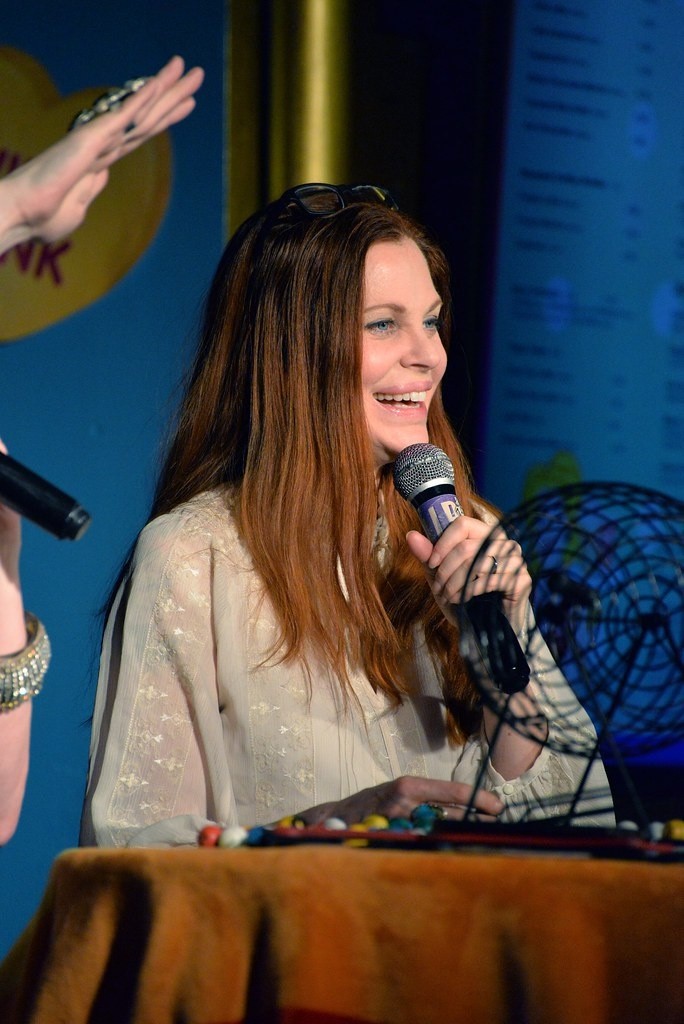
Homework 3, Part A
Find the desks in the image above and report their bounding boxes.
[2,834,681,1023]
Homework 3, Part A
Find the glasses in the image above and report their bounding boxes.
[252,182,400,265]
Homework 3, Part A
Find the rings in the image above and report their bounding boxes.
[490,554,498,576]
[67,74,151,139]
[424,800,446,821]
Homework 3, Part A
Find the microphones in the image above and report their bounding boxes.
[393,442,532,694]
[1,451,89,542]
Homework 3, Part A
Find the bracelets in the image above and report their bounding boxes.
[0,607,53,713]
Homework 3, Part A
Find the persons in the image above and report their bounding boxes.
[68,179,619,845]
[0,46,211,852]
